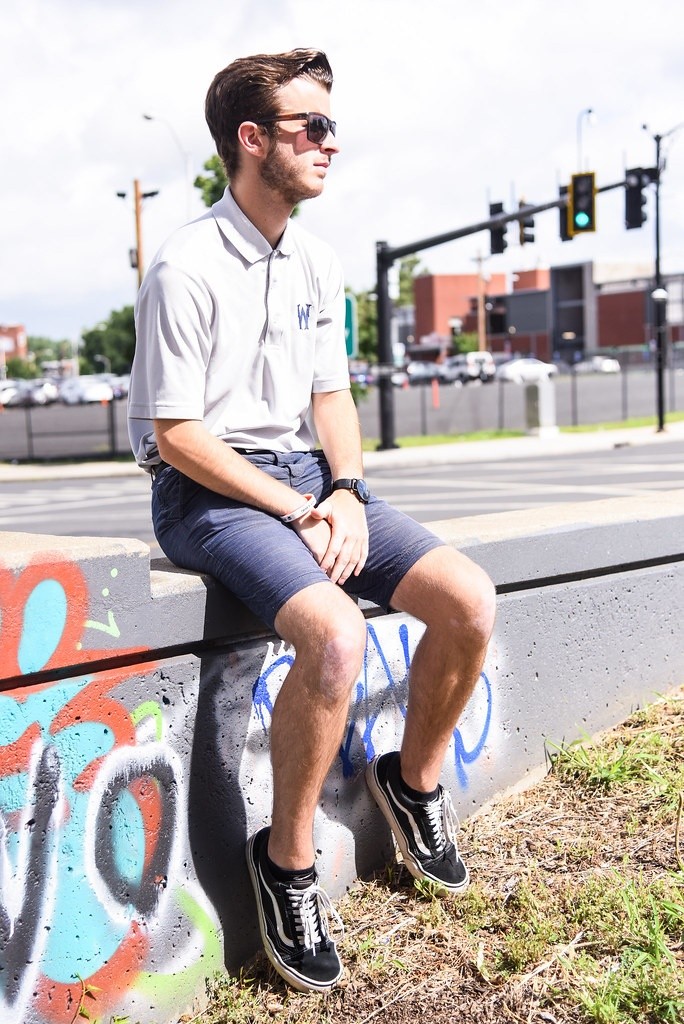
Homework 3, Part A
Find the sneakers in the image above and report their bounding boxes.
[366,750,470,894]
[247,825,345,994]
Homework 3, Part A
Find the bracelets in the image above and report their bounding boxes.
[281,493,317,522]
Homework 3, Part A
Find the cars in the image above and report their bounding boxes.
[0,373,130,404]
[393,353,560,384]
[572,356,620,375]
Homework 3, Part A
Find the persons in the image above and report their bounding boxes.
[126,47,498,995]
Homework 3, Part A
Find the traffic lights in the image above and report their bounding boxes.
[571,173,595,232]
[519,202,535,244]
[626,167,648,228]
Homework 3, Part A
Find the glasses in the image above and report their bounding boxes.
[252,113,336,145]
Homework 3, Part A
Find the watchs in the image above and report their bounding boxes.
[331,478,370,504]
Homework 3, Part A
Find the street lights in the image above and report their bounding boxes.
[117,181,157,285]
